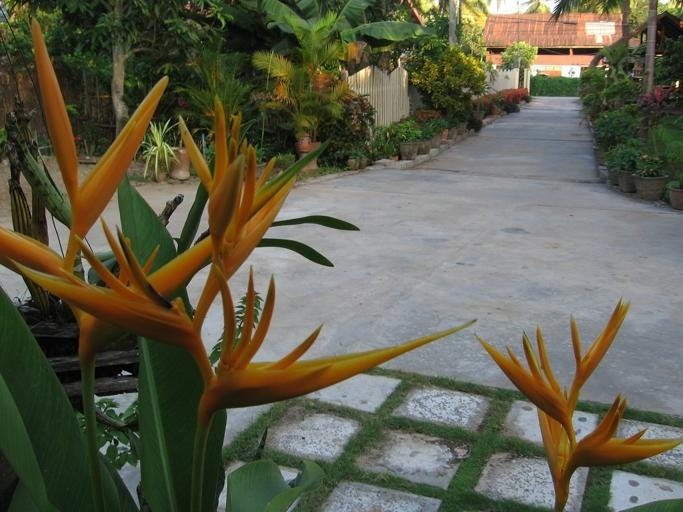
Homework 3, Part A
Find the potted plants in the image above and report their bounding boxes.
[345,110,468,173]
[592,143,683,210]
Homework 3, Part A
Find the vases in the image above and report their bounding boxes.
[295,141,321,173]
[169,149,192,180]
